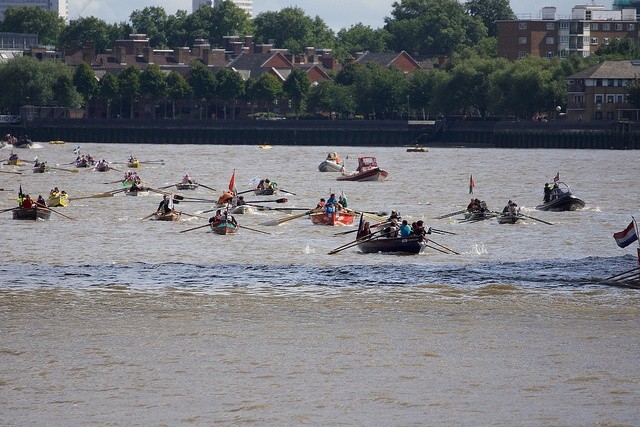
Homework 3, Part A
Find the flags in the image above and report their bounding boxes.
[228,172,235,191]
[469,175,475,195]
[554,172,560,182]
[612,219,639,248]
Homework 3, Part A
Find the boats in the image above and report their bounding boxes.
[211,220,238,234]
[127,162,140,168]
[16,139,32,148]
[356,212,426,255]
[33,164,45,172]
[535,181,586,210]
[50,140,65,144]
[158,211,182,221]
[233,204,252,213]
[256,188,278,195]
[318,160,344,172]
[465,210,497,220]
[99,165,108,172]
[406,149,427,152]
[76,161,86,167]
[175,183,197,190]
[8,159,21,166]
[260,144,272,148]
[47,195,69,207]
[310,212,354,226]
[125,186,145,196]
[337,156,388,181]
[498,216,525,224]
[13,206,51,219]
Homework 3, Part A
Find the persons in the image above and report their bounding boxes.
[158,195,172,214]
[183,174,192,184]
[265,178,271,189]
[360,221,375,241]
[49,186,67,198]
[552,182,560,199]
[399,220,412,239]
[209,209,239,229]
[544,183,551,203]
[220,190,247,208]
[76,154,93,165]
[412,220,426,240]
[17,194,26,206]
[412,222,417,231]
[316,192,345,212]
[256,179,264,190]
[383,220,400,237]
[97,159,108,167]
[123,170,141,187]
[386,209,402,224]
[325,151,340,165]
[127,155,136,164]
[21,194,33,209]
[36,194,45,207]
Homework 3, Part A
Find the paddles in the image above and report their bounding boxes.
[69,193,112,200]
[259,212,311,226]
[172,200,215,204]
[173,194,218,203]
[354,211,387,222]
[47,165,79,173]
[244,198,289,203]
